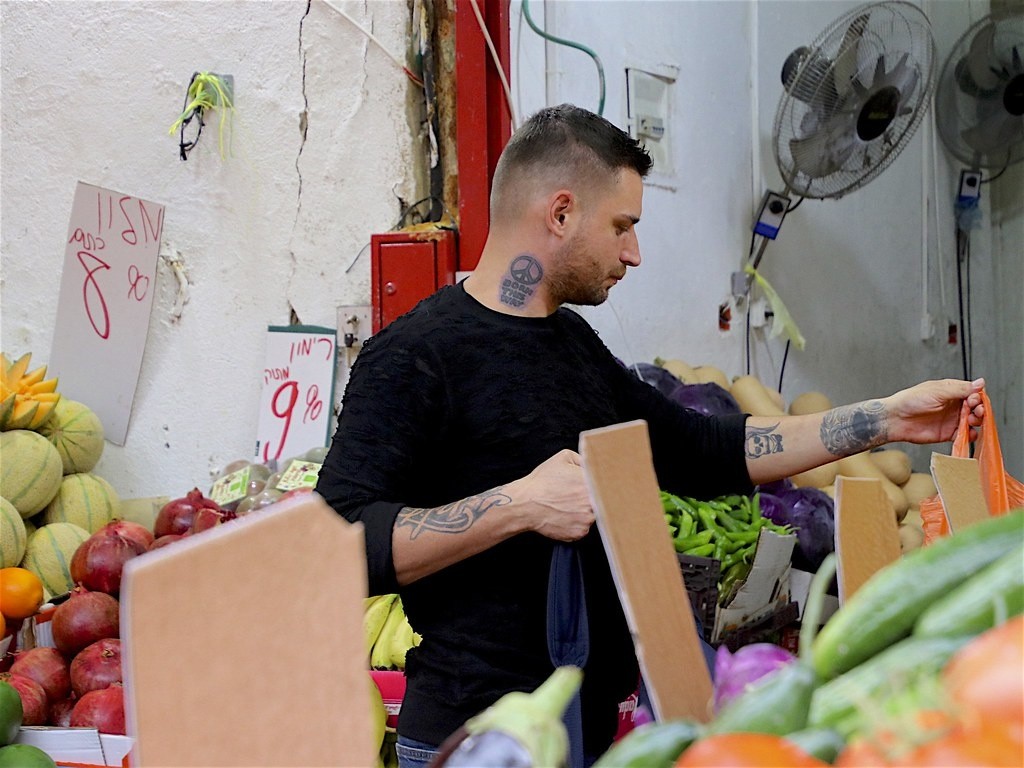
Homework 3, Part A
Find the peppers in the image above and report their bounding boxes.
[657,489,802,604]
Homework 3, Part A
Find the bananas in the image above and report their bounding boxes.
[363,593,423,768]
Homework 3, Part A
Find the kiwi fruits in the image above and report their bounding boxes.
[208,446,330,512]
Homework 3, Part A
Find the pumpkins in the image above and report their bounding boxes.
[655,355,938,553]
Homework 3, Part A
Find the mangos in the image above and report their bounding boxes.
[0,680,57,768]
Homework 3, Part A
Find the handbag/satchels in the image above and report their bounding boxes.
[921,388,1024,547]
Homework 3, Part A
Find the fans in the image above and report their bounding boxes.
[746,1,938,243]
[934,4,1024,215]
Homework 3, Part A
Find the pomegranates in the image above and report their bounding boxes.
[0,486,314,736]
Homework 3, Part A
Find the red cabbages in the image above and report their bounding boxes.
[629,362,836,573]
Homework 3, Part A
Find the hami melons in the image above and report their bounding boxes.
[0,351,122,603]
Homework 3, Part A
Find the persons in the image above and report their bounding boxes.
[308,96,988,768]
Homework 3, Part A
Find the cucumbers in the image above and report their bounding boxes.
[590,501,1024,767]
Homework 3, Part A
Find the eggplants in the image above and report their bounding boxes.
[424,663,586,768]
